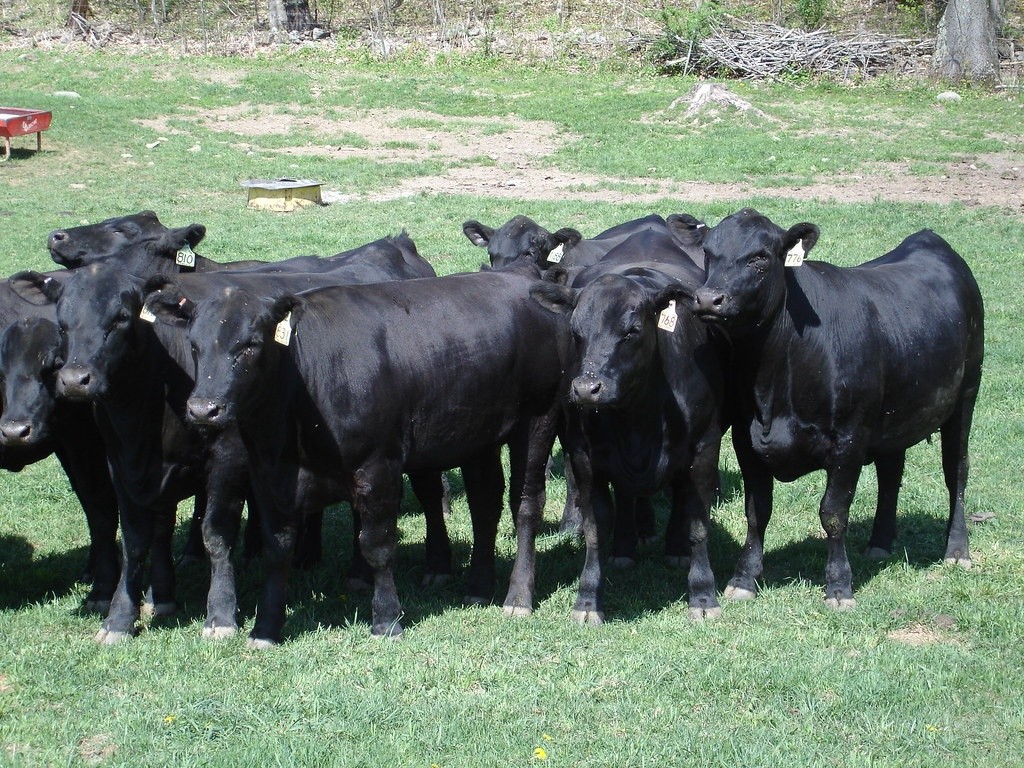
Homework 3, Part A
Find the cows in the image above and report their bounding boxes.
[0,205,988,654]
